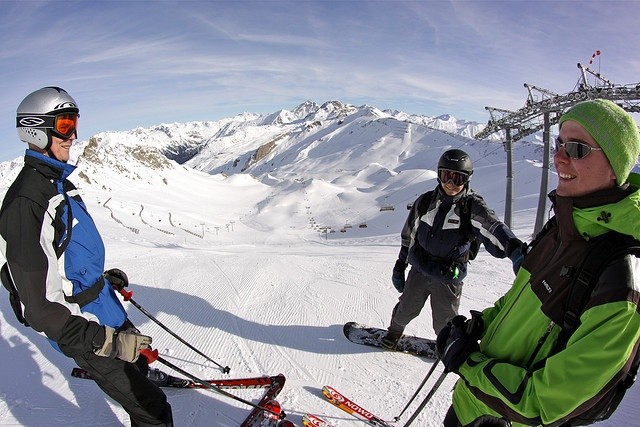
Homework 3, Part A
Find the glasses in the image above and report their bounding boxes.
[17,112,79,140]
[437,168,473,186]
[555,138,602,159]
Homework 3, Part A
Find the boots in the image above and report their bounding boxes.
[372,328,403,349]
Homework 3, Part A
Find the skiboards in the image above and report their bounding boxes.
[301,384,395,427]
[71,367,286,427]
[259,399,296,427]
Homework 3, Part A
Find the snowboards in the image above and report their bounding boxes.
[343,321,438,358]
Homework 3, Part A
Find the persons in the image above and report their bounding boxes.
[436,99,640,427]
[0,87,174,427]
[381,149,528,349]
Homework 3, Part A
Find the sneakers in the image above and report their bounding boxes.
[146,368,169,386]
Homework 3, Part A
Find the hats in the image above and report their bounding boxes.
[558,97,640,186]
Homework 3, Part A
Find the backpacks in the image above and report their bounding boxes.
[528,214,640,426]
[417,190,481,259]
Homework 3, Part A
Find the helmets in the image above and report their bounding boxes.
[437,149,473,203]
[17,86,79,150]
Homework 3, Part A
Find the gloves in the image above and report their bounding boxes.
[104,267,129,289]
[437,324,471,373]
[92,323,153,363]
[509,244,525,275]
[449,309,485,336]
[392,269,405,292]
